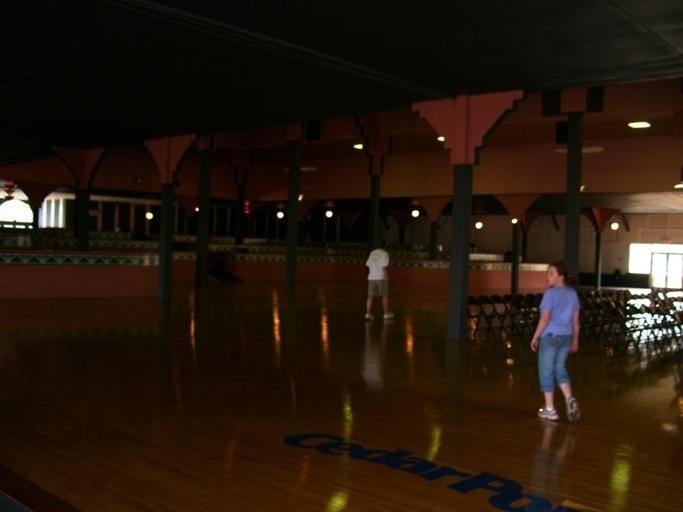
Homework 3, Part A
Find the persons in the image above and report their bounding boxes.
[527,259,583,427]
[361,318,392,401]
[361,239,394,321]
[527,417,580,502]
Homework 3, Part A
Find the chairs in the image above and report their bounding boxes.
[468,287,683,359]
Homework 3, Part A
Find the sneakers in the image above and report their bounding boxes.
[565,397,581,422]
[538,405,559,420]
[364,313,394,320]
